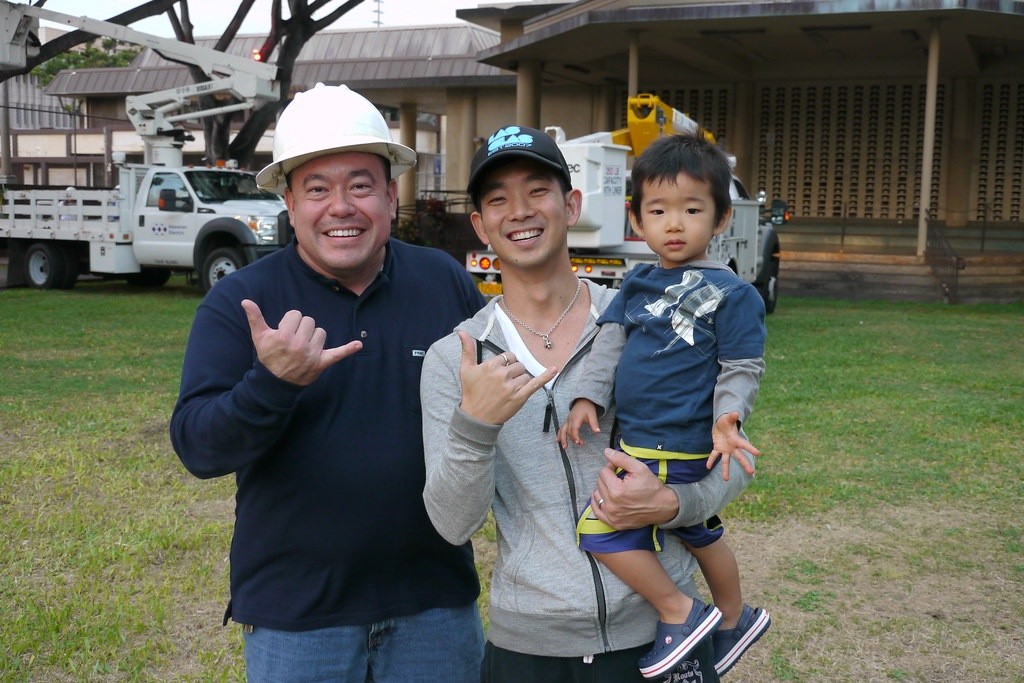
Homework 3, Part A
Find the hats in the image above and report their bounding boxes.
[466,125,572,196]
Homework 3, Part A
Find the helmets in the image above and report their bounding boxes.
[255,83,417,196]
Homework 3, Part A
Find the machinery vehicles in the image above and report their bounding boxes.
[462,92,790,316]
[0,0,291,299]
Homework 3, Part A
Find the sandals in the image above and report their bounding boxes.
[712,603,771,679]
[638,597,724,681]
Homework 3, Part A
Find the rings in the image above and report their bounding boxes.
[500,353,509,366]
[598,499,603,507]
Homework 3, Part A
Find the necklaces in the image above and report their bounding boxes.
[501,275,581,349]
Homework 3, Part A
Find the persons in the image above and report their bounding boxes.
[421,127,756,683]
[555,134,771,676]
[170,83,490,683]
